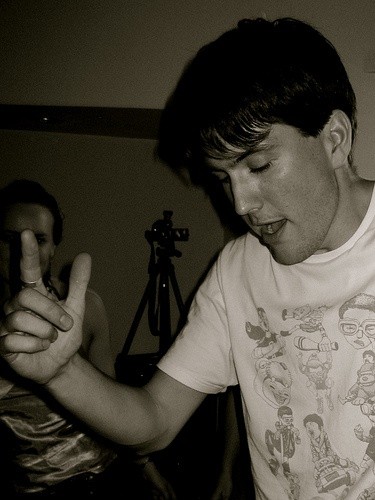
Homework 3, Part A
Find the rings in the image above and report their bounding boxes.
[20,275,43,288]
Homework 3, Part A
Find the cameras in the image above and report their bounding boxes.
[145,209,190,242]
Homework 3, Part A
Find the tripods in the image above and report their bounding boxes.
[116,242,183,386]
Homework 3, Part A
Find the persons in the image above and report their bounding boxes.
[0,16,374,500]
